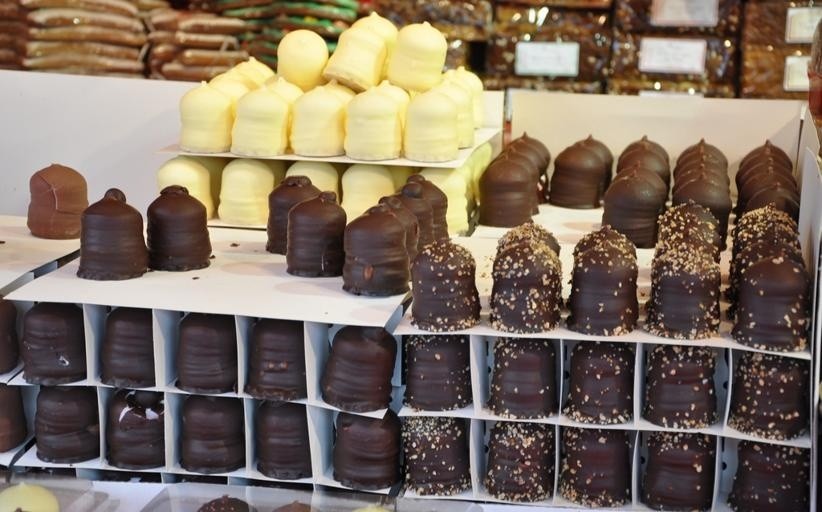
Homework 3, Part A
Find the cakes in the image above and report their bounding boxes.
[727,439,810,512]
[321,26,387,94]
[484,337,559,419]
[243,318,307,400]
[0,383,28,453]
[617,144,671,186]
[22,301,87,386]
[206,68,250,105]
[654,229,720,258]
[147,185,213,272]
[287,192,346,278]
[736,145,798,180]
[438,77,475,149]
[459,157,476,212]
[350,10,399,80]
[343,205,410,297]
[403,335,473,412]
[478,152,531,228]
[196,495,259,512]
[0,294,20,374]
[156,156,214,219]
[175,312,238,394]
[28,164,89,240]
[178,395,246,474]
[419,168,469,236]
[562,340,635,425]
[272,500,321,512]
[319,325,397,413]
[721,238,811,320]
[402,86,458,163]
[640,431,715,512]
[641,343,720,430]
[385,21,448,93]
[376,80,410,130]
[340,163,396,226]
[105,389,165,470]
[732,166,800,214]
[266,175,323,255]
[232,56,275,86]
[322,78,357,96]
[470,142,493,207]
[642,242,721,340]
[670,155,734,186]
[0,481,60,512]
[34,385,100,464]
[288,86,344,157]
[410,237,482,333]
[97,307,155,388]
[672,163,731,196]
[77,187,150,281]
[482,421,555,503]
[391,182,434,253]
[489,236,564,335]
[505,132,550,170]
[352,504,396,512]
[285,161,340,205]
[343,86,404,161]
[656,200,721,226]
[496,146,547,215]
[576,135,614,200]
[677,139,732,168]
[259,77,305,103]
[557,427,631,509]
[377,196,418,263]
[179,81,237,154]
[443,66,483,130]
[601,169,666,248]
[736,158,798,190]
[331,408,401,491]
[673,144,728,176]
[254,399,312,480]
[217,158,275,226]
[229,84,291,156]
[654,212,723,247]
[548,142,602,208]
[566,239,638,336]
[672,173,732,251]
[618,135,669,162]
[400,417,472,497]
[190,156,230,209]
[574,223,636,257]
[738,140,792,171]
[496,220,561,256]
[276,29,329,94]
[727,350,810,441]
[407,175,448,241]
[385,166,421,194]
[507,138,550,204]
[734,201,799,233]
[732,213,801,255]
[257,160,287,189]
[730,248,814,351]
[613,158,669,202]
[731,182,800,225]
